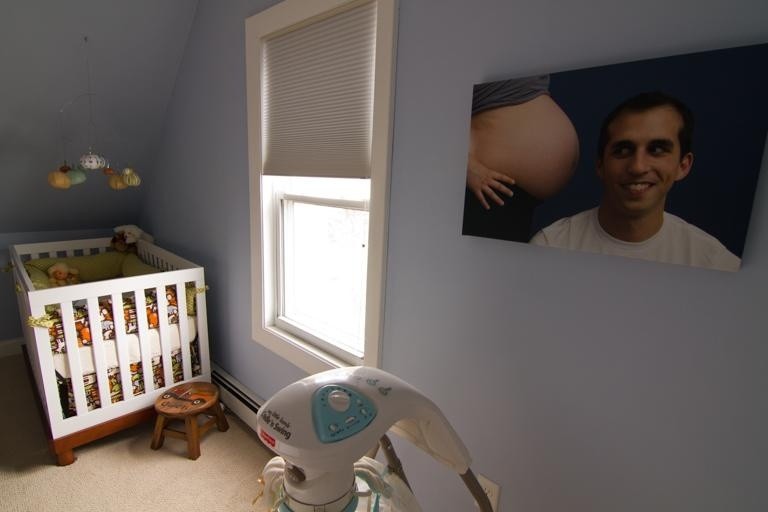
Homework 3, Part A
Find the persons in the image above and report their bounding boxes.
[464,73,583,241]
[527,92,744,272]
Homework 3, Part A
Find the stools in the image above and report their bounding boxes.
[150,382,229,460]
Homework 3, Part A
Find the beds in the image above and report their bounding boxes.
[9,235,212,466]
